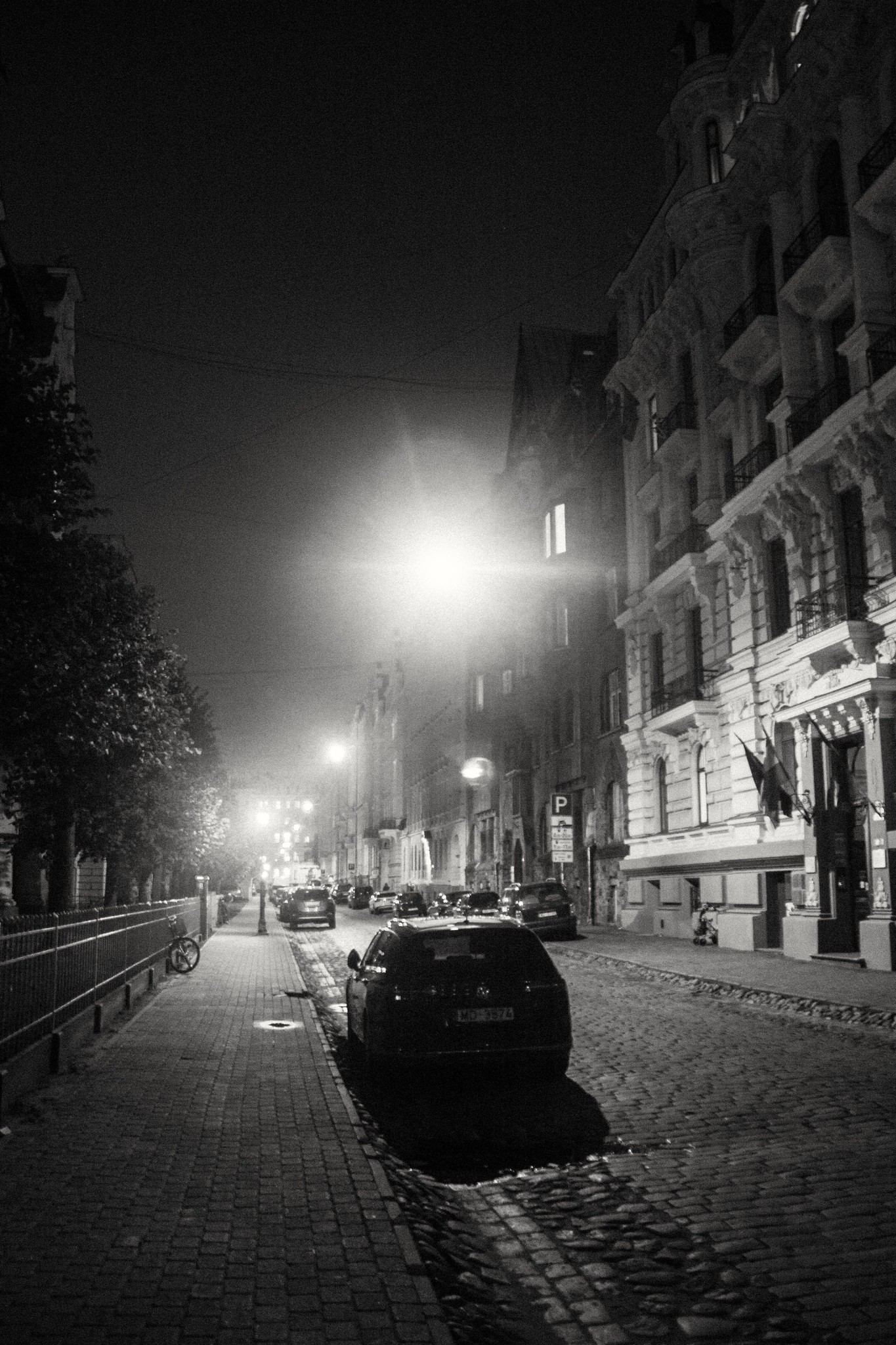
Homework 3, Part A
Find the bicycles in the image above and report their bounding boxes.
[164,912,200,973]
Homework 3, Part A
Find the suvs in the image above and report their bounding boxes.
[346,914,573,1088]
[495,873,577,941]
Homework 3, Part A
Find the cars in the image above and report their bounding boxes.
[269,879,501,930]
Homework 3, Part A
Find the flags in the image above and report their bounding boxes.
[742,736,794,829]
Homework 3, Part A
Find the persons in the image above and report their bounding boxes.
[382,883,390,892]
